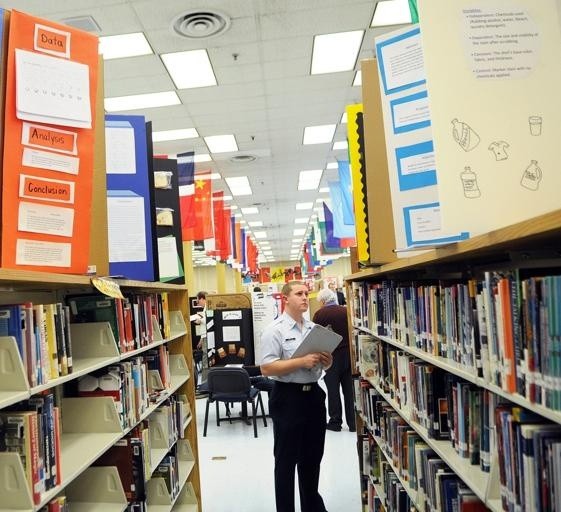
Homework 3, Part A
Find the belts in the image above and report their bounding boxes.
[274,380,317,391]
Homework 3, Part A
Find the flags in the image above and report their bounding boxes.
[300,139,357,276]
[152,152,259,275]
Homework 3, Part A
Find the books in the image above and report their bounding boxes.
[0,292,185,512]
[350,268,561,512]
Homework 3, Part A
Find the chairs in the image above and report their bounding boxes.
[202,364,269,439]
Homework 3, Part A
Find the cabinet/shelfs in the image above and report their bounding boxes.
[1,255,206,512]
[342,215,560,512]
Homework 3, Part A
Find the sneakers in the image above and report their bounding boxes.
[348,424,357,432]
[325,422,343,432]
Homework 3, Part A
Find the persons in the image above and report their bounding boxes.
[194,291,208,394]
[260,281,356,512]
[254,287,261,292]
